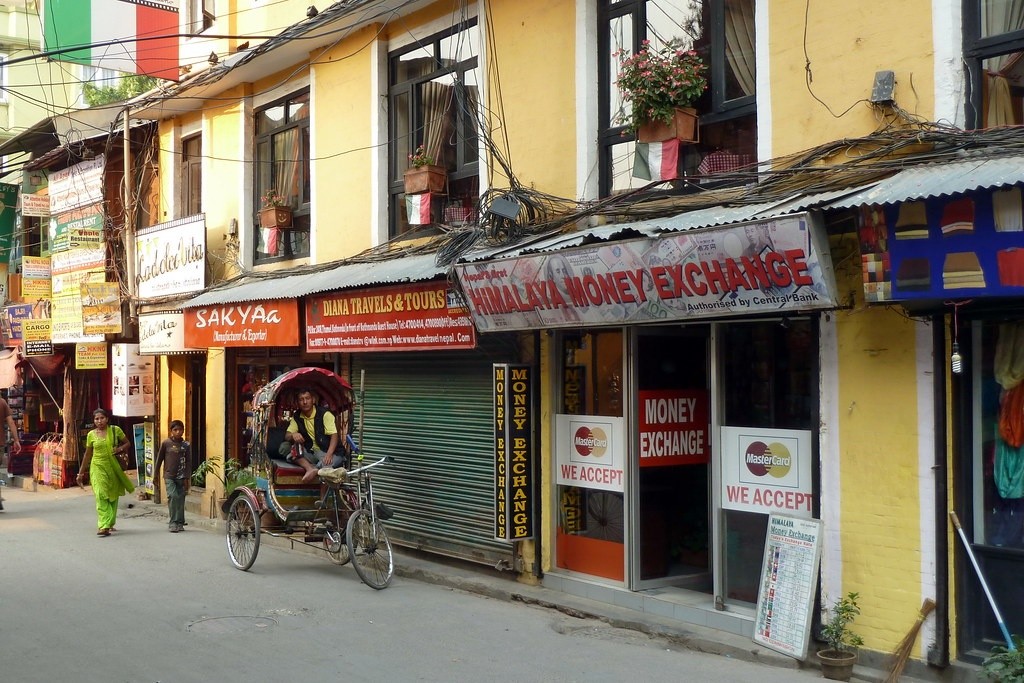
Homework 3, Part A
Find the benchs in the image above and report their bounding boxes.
[263,427,350,510]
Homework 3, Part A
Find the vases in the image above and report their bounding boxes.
[635,105,698,144]
[403,165,447,195]
[258,208,291,228]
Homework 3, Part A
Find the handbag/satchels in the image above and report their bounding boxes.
[112,425,129,471]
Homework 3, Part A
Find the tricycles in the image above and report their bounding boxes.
[220,367,396,591]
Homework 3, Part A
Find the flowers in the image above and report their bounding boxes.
[610,41,708,137]
[260,188,285,208]
[408,144,432,168]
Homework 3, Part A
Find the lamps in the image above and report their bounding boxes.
[29,174,42,187]
[546,329,553,337]
[83,148,96,160]
[780,316,790,329]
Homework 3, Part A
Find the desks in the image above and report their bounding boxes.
[445,208,476,224]
[698,154,752,177]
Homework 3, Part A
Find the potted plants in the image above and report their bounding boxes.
[816,591,864,680]
[194,456,276,532]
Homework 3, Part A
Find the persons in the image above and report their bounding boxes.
[76,408,134,536]
[153,420,192,533]
[279,387,346,509]
[0,397,22,511]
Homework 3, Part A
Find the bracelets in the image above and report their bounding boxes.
[78,473,85,476]
[119,447,123,451]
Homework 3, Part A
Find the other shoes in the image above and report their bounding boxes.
[286,506,300,512]
[109,527,116,531]
[97,528,112,536]
[178,523,184,530]
[314,501,327,509]
[169,527,178,531]
